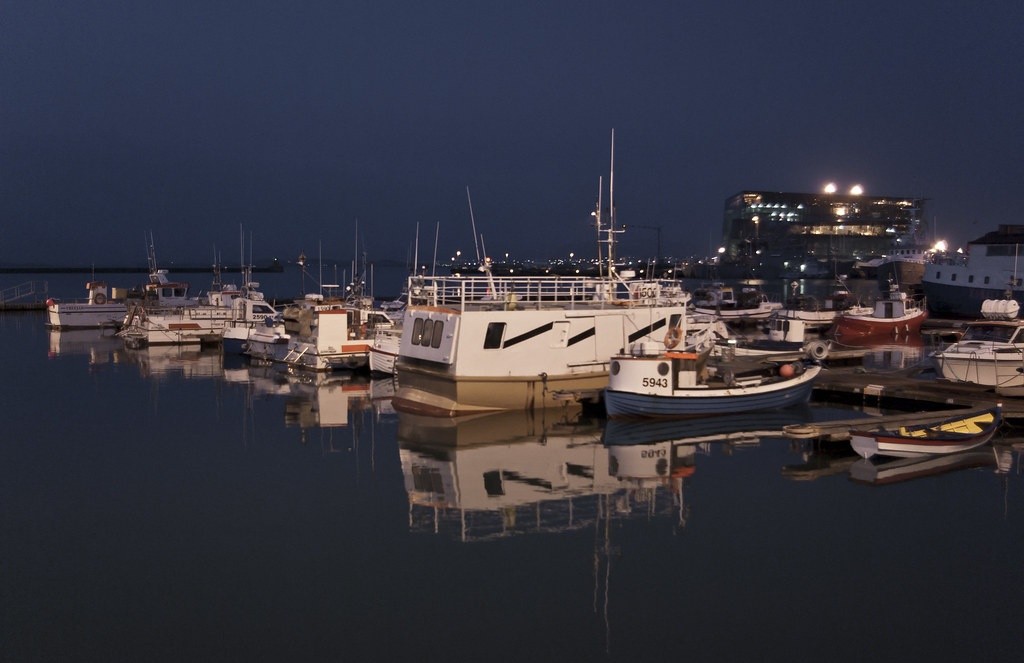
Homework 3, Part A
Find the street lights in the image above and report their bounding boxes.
[791,281,799,293]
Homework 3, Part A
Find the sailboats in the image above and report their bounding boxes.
[296,249,310,271]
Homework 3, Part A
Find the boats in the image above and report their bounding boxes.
[933,299,1024,399]
[224,298,285,357]
[378,292,409,321]
[491,264,523,276]
[847,403,1004,462]
[46,230,209,331]
[922,249,1024,318]
[282,366,370,454]
[243,299,394,371]
[391,129,717,417]
[122,341,202,381]
[849,434,1024,487]
[773,295,874,333]
[365,374,400,424]
[245,357,292,397]
[860,249,928,301]
[824,284,927,347]
[397,411,698,654]
[116,281,265,346]
[687,282,781,324]
[686,314,729,343]
[449,266,476,275]
[366,329,404,374]
[48,329,129,367]
[604,342,832,419]
[784,446,851,480]
[222,354,251,383]
